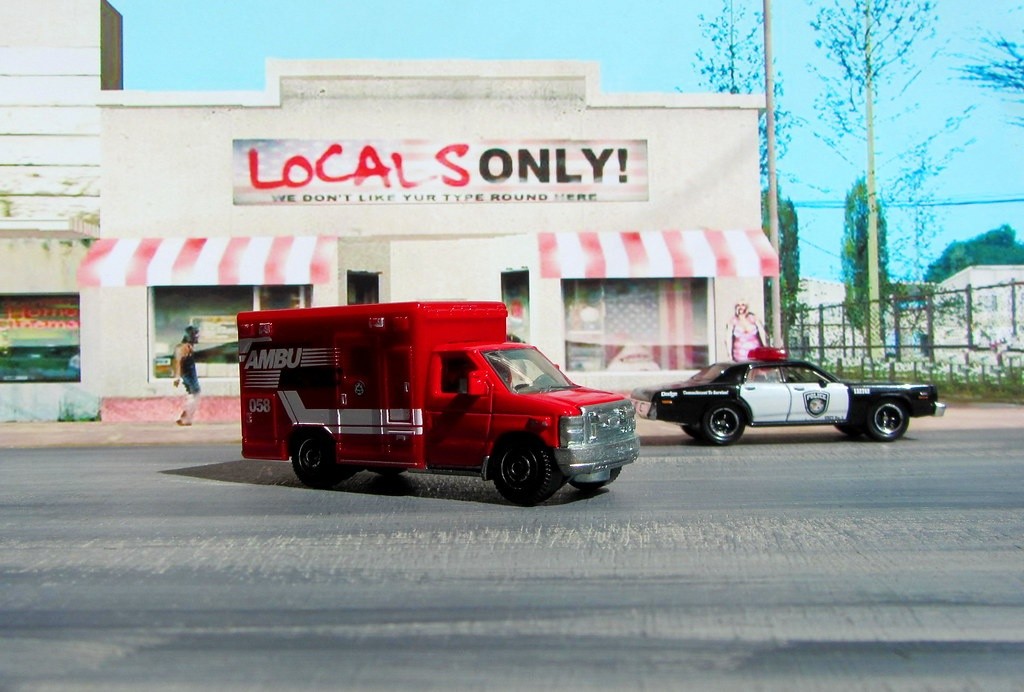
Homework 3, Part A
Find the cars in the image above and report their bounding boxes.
[631,342,948,447]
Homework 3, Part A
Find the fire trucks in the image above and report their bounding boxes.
[236,300,641,508]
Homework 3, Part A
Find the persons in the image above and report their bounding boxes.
[173,325,202,426]
